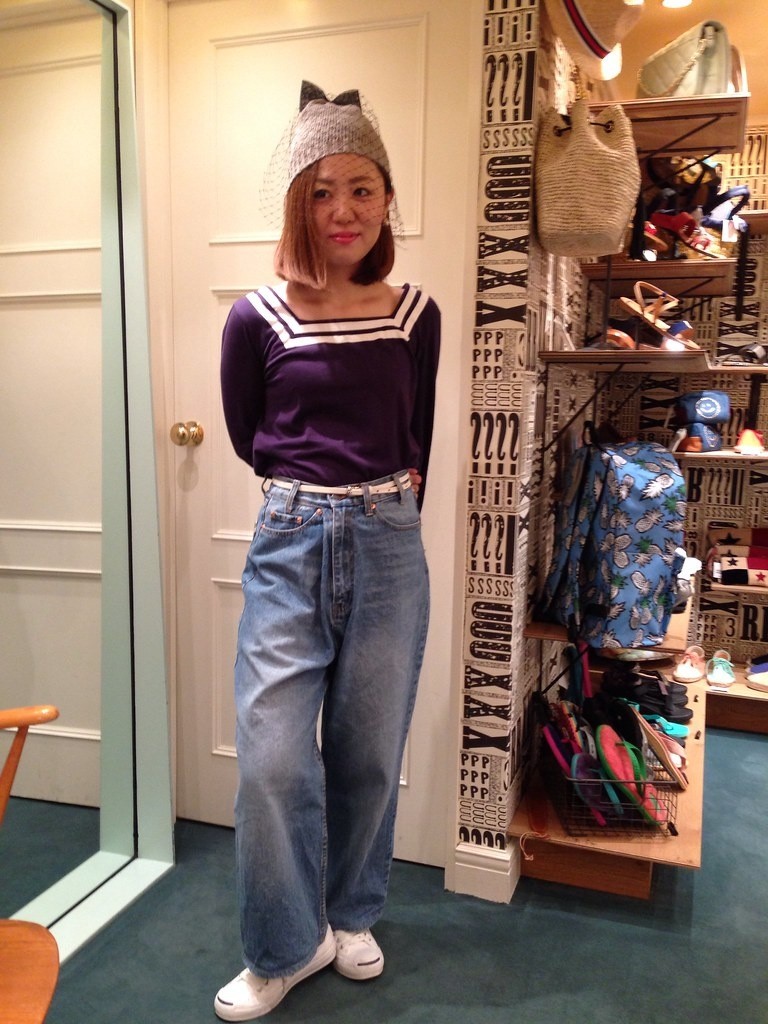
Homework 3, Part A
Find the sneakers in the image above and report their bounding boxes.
[674,646,705,682]
[707,649,736,687]
[331,927,384,981]
[214,922,337,1021]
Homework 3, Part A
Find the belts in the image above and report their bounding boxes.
[272,472,413,496]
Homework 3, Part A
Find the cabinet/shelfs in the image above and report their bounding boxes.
[506,92,768,900]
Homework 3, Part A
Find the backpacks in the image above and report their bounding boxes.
[538,417,686,651]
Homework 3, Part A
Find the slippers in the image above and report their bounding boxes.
[529,665,692,830]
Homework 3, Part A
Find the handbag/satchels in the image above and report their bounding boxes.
[634,20,747,97]
[533,99,639,258]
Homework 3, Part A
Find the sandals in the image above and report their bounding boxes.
[607,280,702,350]
[649,208,724,259]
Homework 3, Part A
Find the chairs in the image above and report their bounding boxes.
[0,705,60,1023]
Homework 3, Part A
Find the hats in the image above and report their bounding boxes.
[285,81,389,188]
[544,0,641,81]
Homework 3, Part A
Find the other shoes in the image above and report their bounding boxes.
[635,154,751,259]
[744,654,768,692]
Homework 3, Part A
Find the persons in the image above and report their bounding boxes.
[213,78,441,1022]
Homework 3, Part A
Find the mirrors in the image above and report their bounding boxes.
[0,0,176,965]
